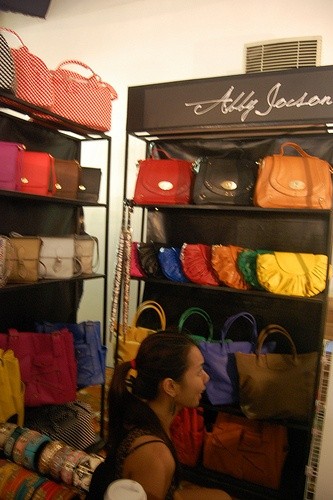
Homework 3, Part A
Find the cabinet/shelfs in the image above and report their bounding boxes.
[0,89,333,500]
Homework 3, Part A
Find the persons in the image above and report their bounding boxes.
[106,331,231,500]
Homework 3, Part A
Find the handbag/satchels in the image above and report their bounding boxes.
[130,240,329,298]
[0,34,16,96]
[33,60,117,132]
[133,144,195,205]
[112,300,320,489]
[0,140,102,203]
[0,230,100,285]
[0,318,109,448]
[191,149,258,206]
[0,27,54,110]
[252,143,333,210]
